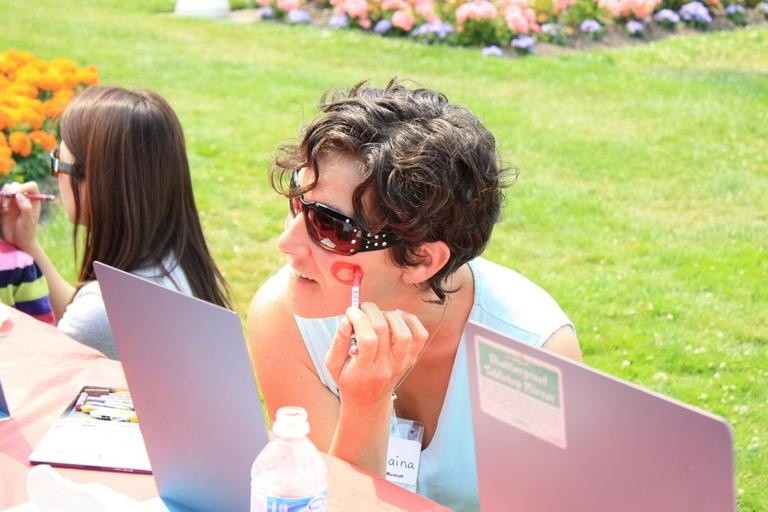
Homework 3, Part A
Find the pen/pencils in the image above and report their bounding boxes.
[349,265,362,355]
[0,192,55,202]
[76,389,140,423]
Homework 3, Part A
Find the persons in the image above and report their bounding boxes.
[0,82,239,365]
[0,238,58,329]
[243,72,589,512]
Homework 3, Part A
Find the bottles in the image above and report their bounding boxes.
[249,406,329,512]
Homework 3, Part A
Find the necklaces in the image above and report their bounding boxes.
[386,270,455,403]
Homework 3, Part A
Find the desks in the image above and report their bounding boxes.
[0,303,455,512]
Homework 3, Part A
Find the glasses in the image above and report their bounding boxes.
[49,147,84,180]
[287,169,403,257]
